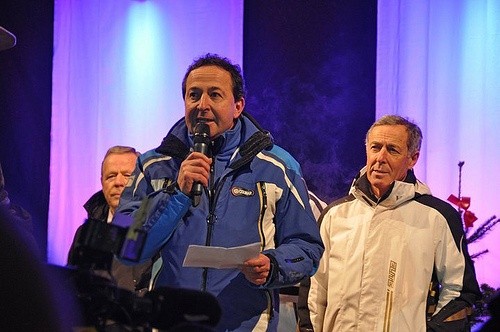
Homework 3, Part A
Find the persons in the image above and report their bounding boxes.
[111,53,325,332]
[68,146,161,293]
[296,115,481,332]
[0,167,73,332]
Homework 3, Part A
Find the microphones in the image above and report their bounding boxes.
[190,123,211,208]
